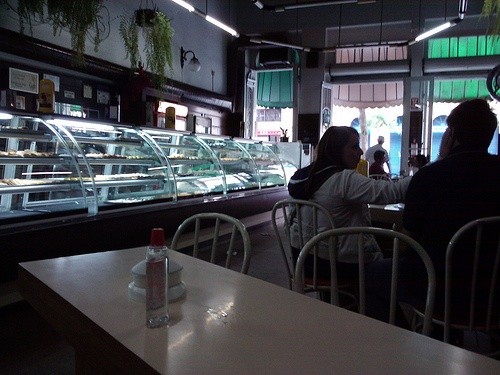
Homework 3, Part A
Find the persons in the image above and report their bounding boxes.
[365,136,391,174]
[414,154,431,169]
[283,126,415,284]
[369,150,390,180]
[365,98,500,345]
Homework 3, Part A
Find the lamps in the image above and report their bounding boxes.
[170,0,468,71]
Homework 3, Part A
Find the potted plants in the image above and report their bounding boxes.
[45,0,111,74]
[109,0,177,103]
[4,0,45,39]
[279,128,288,142]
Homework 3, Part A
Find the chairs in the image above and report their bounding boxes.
[170,200,500,360]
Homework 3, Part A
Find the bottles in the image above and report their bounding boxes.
[146,227,169,328]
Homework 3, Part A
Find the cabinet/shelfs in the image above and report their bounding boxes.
[0,130,300,219]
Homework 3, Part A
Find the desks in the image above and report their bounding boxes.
[18,247,500,375]
[366,203,405,228]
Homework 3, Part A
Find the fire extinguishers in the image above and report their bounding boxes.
[411,138,419,158]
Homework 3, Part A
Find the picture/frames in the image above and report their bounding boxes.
[94,88,111,105]
[107,102,120,122]
[41,72,61,93]
[82,83,92,100]
